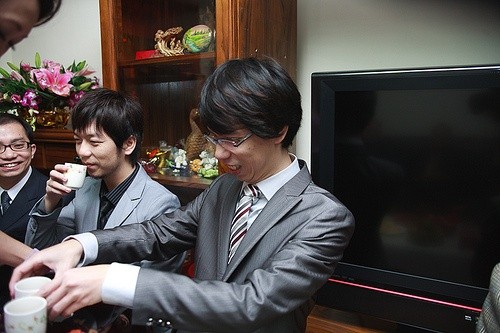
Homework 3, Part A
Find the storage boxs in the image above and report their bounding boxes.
[135,49,156,60]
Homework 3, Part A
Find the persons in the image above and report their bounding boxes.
[25,87,185,333]
[0,0,63,279]
[0,113,64,294]
[10,54,355,332]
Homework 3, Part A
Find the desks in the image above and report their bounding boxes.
[304,304,403,333]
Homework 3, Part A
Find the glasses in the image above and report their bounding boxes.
[0,141,32,154]
[203,130,253,148]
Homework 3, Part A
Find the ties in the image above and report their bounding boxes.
[227,184,262,266]
[98,196,112,230]
[1,190,11,214]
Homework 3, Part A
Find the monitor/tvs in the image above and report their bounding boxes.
[310,64,500,333]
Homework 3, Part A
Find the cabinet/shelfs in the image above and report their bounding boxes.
[99,0,299,190]
[29,129,78,177]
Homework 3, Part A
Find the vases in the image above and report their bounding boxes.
[17,107,72,131]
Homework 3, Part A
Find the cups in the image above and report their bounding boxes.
[4,296,47,333]
[61,162,88,191]
[15,276,52,298]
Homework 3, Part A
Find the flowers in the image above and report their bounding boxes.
[0,51,101,117]
